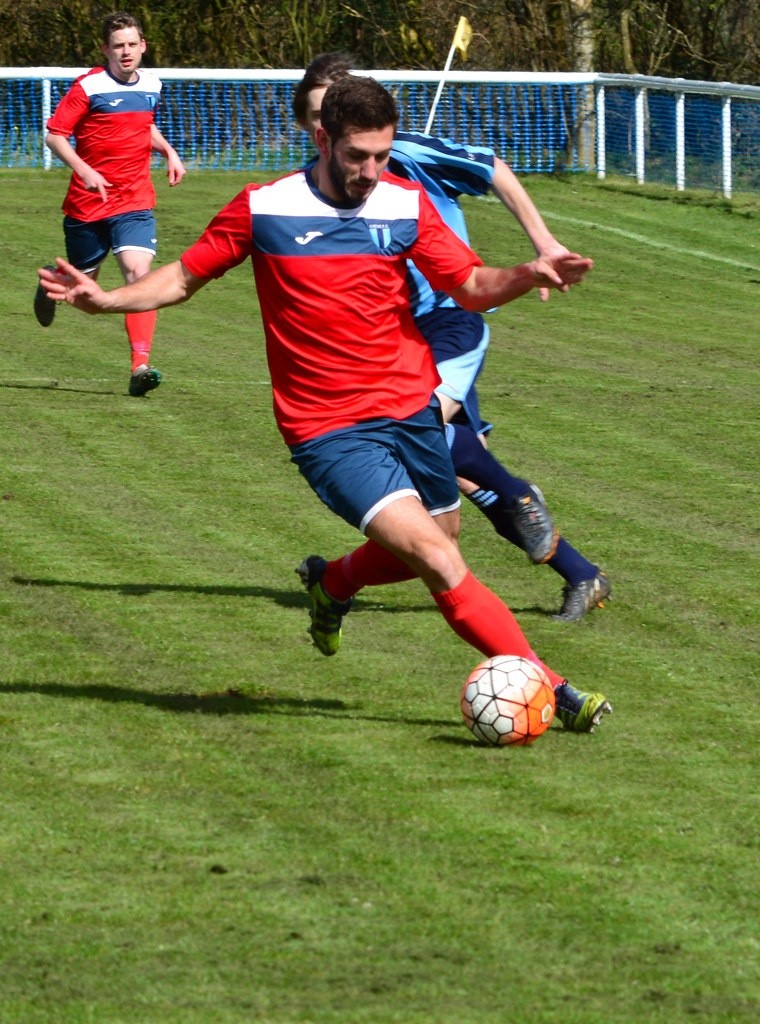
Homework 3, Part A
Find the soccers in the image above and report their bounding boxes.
[459,656,558,748]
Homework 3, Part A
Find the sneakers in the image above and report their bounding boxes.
[32,265,56,327]
[502,477,559,566]
[552,678,613,734]
[295,553,357,658]
[128,366,162,396]
[552,565,613,621]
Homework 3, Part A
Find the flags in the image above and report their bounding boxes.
[454,16,473,60]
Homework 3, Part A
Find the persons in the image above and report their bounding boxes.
[35,74,613,735]
[290,52,614,620]
[32,12,185,396]
